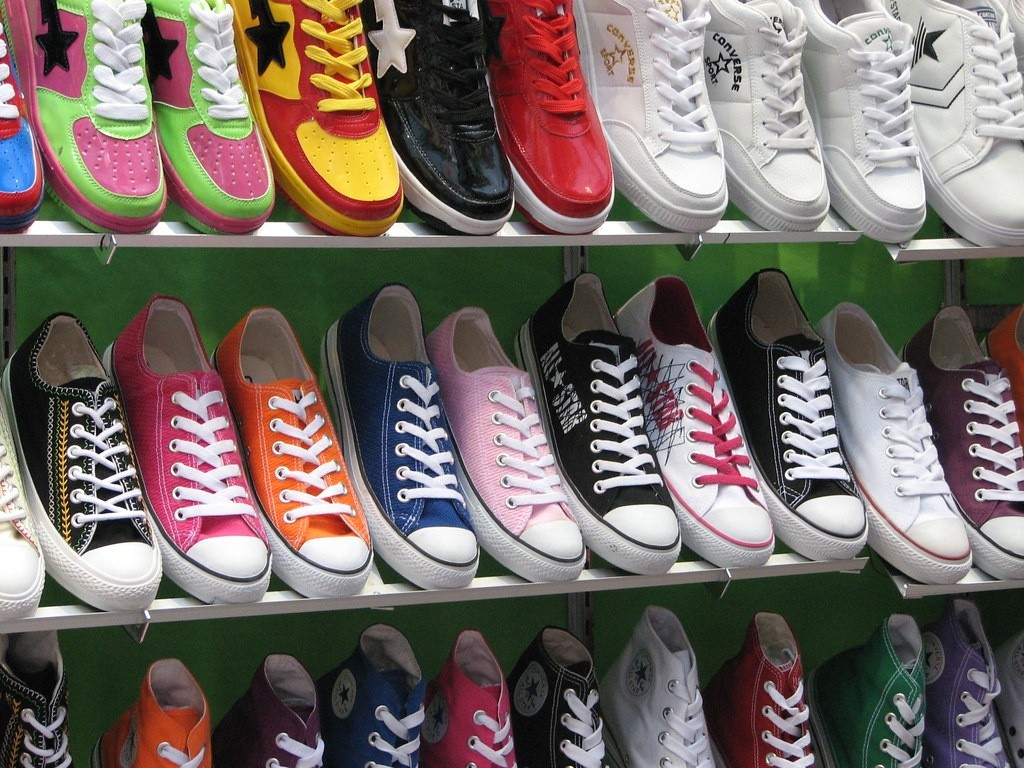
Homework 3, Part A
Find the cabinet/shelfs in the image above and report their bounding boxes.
[0,0,1024,768]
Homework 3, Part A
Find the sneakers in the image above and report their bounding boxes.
[0,0,1022,239]
[0,268,1024,634]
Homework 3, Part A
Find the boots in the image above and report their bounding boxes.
[0,600,1023,768]
